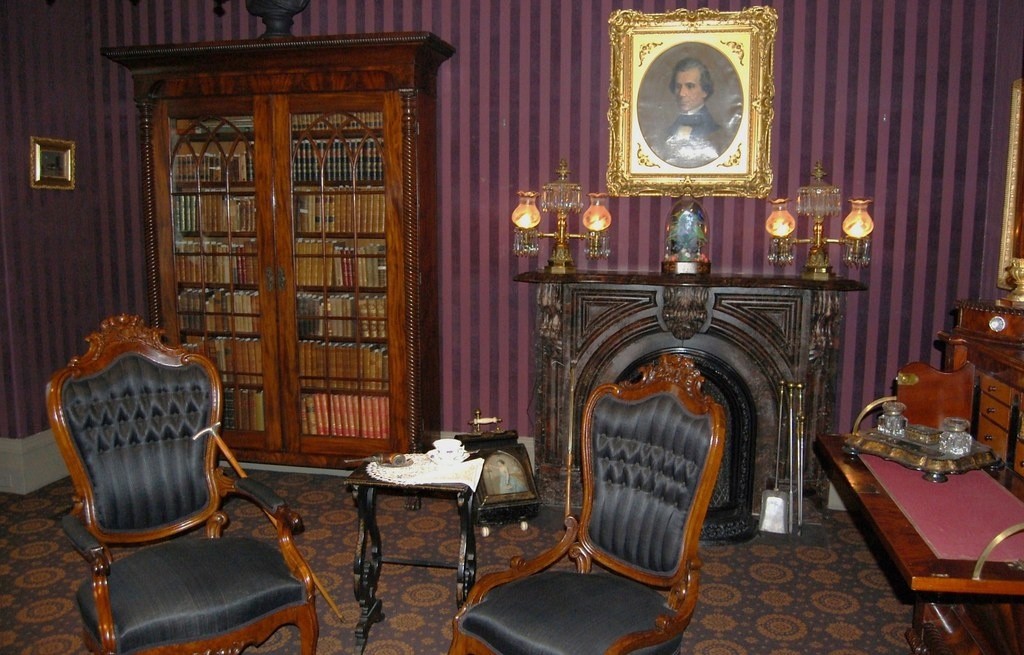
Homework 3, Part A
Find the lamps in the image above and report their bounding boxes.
[512,159,613,272]
[765,161,874,282]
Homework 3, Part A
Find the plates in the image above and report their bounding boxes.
[427,449,470,465]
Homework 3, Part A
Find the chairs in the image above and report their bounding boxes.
[448,352,726,655]
[45,312,319,655]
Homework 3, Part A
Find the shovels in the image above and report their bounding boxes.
[758,379,788,536]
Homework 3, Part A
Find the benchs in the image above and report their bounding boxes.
[812,395,1024,655]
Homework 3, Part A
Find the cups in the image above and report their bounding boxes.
[432,439,465,459]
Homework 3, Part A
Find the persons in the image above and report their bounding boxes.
[666,58,725,168]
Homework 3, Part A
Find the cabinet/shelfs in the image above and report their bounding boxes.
[102,31,455,469]
[937,297,1024,506]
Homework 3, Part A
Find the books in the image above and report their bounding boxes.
[177,288,259,331]
[298,293,386,337]
[220,386,263,431]
[172,151,254,182]
[174,116,254,132]
[174,238,258,284]
[301,393,390,440]
[184,335,262,387]
[293,186,384,232]
[176,195,255,233]
[292,113,382,129]
[292,138,384,181]
[297,341,388,390]
[295,236,387,289]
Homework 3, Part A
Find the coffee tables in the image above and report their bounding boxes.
[344,446,488,653]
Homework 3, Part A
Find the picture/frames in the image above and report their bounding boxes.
[606,2,778,199]
[997,80,1024,291]
[30,136,76,190]
